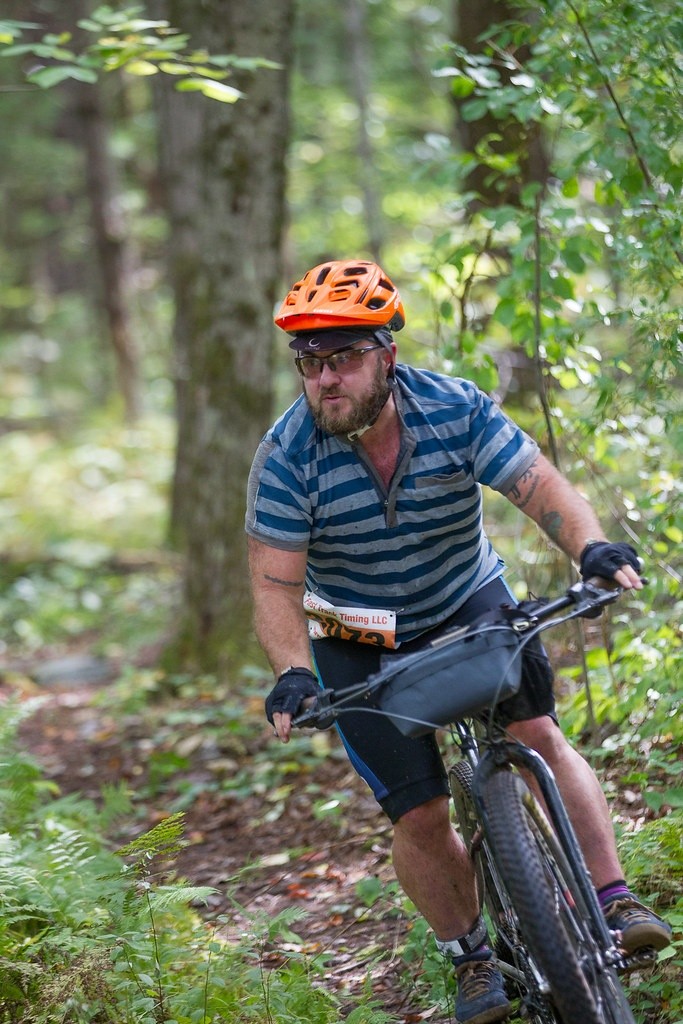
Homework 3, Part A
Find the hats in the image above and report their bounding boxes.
[288,332,393,352]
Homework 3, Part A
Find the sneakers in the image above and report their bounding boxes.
[451,949,513,1024]
[602,891,671,952]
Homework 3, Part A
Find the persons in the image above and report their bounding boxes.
[244,260,672,1023]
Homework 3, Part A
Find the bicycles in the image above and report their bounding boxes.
[275,551,661,1023]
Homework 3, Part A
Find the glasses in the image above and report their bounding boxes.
[294,345,380,378]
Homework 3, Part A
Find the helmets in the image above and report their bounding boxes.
[274,259,406,335]
